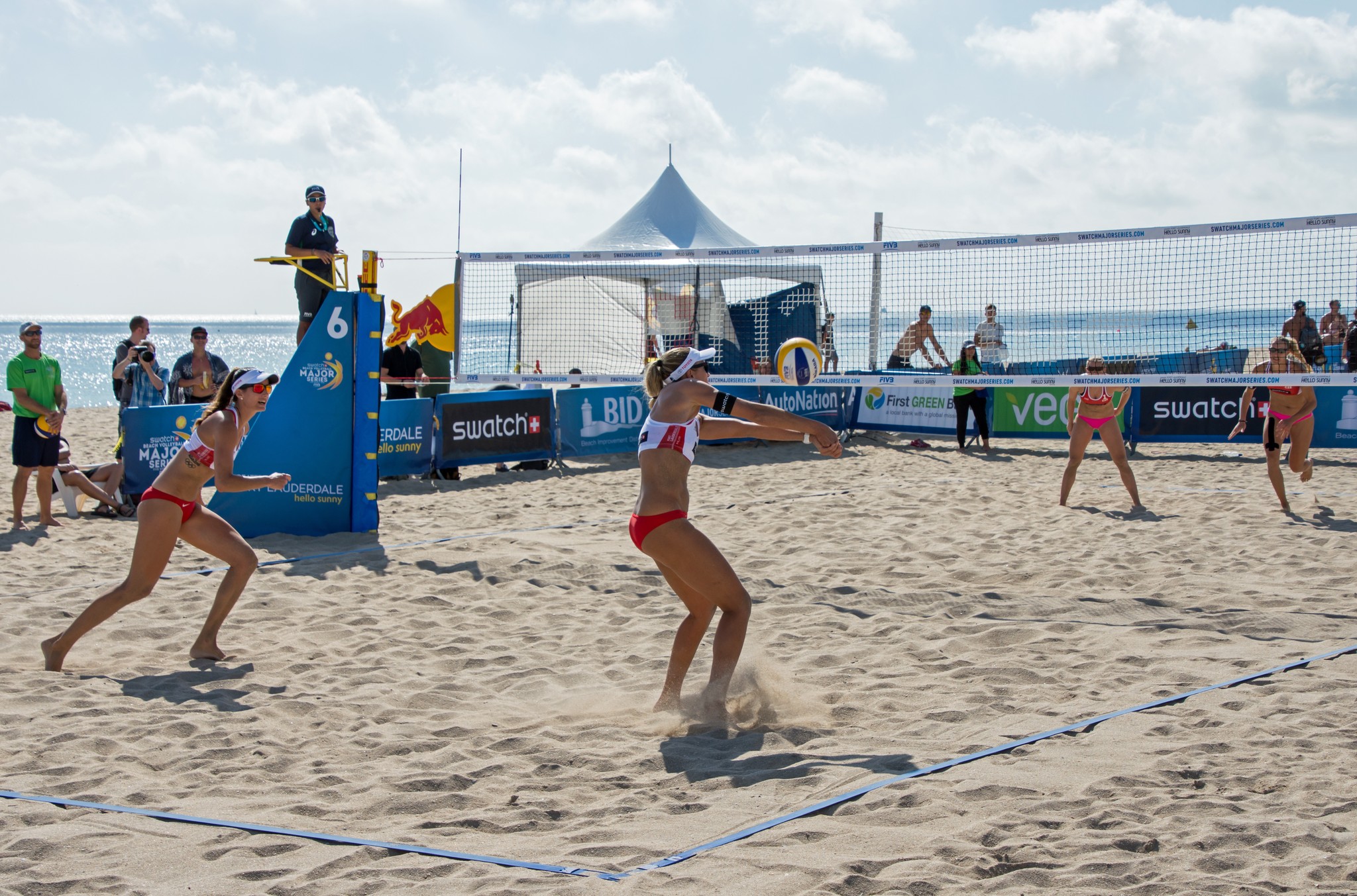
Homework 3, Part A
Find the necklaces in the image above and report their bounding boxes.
[193,350,205,362]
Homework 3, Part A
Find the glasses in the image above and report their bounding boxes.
[1336,304,1341,307]
[1269,347,1290,353]
[1087,366,1103,372]
[307,196,326,203]
[25,330,42,336]
[240,384,272,394]
[192,335,206,339]
[692,362,709,373]
[920,311,930,316]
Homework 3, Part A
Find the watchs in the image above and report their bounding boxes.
[400,379,404,386]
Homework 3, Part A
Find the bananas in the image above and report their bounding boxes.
[200,371,209,389]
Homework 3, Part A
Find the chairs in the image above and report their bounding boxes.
[30,463,123,517]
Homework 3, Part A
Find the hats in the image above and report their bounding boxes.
[231,369,280,395]
[306,185,326,198]
[827,312,835,318]
[919,306,932,312]
[961,340,977,348]
[191,326,208,337]
[19,320,42,335]
[1314,353,1328,367]
[1294,300,1306,311]
[663,347,716,385]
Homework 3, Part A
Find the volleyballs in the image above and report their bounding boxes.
[34,415,56,439]
[774,337,822,386]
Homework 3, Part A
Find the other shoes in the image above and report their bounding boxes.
[495,464,509,472]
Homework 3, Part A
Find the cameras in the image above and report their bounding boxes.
[133,345,155,362]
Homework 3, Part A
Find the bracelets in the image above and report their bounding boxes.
[60,407,67,415]
[1238,420,1247,422]
[311,248,316,256]
[126,356,133,363]
[337,250,340,253]
[802,433,812,444]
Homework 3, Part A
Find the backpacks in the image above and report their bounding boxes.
[1299,317,1323,357]
[112,339,137,401]
[1347,320,1357,353]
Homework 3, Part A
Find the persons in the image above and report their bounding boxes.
[1281,299,1357,373]
[974,304,1007,364]
[285,185,344,348]
[41,367,291,672]
[7,316,230,531]
[629,311,843,723]
[952,339,993,452]
[1057,356,1142,507]
[887,306,954,370]
[488,368,582,472]
[380,326,453,412]
[1228,335,1317,511]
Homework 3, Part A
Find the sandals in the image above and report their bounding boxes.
[116,503,135,517]
[91,504,117,518]
